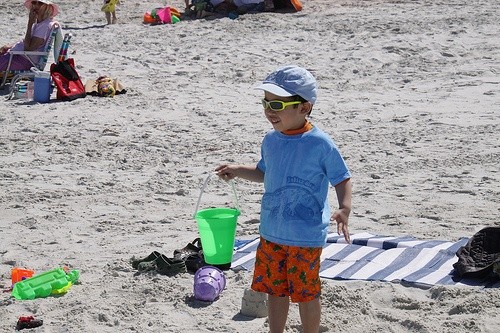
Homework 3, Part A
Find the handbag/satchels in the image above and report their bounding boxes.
[50,58,86,99]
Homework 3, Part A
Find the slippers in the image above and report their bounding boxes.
[133,251,187,273]
[173,238,231,268]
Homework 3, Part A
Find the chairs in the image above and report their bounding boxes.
[5,33,72,100]
[0,23,59,88]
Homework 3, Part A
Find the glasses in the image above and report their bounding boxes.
[31,1,42,4]
[261,100,301,111]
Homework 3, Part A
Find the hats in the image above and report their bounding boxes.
[255,65,316,105]
[24,0,57,17]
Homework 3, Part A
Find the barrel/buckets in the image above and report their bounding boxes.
[193,266,226,301]
[192,172,241,264]
[31,67,51,102]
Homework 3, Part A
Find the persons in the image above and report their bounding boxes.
[213,64,354,333]
[102,0,121,25]
[0,0,58,72]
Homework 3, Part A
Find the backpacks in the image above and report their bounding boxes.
[452,225,500,285]
